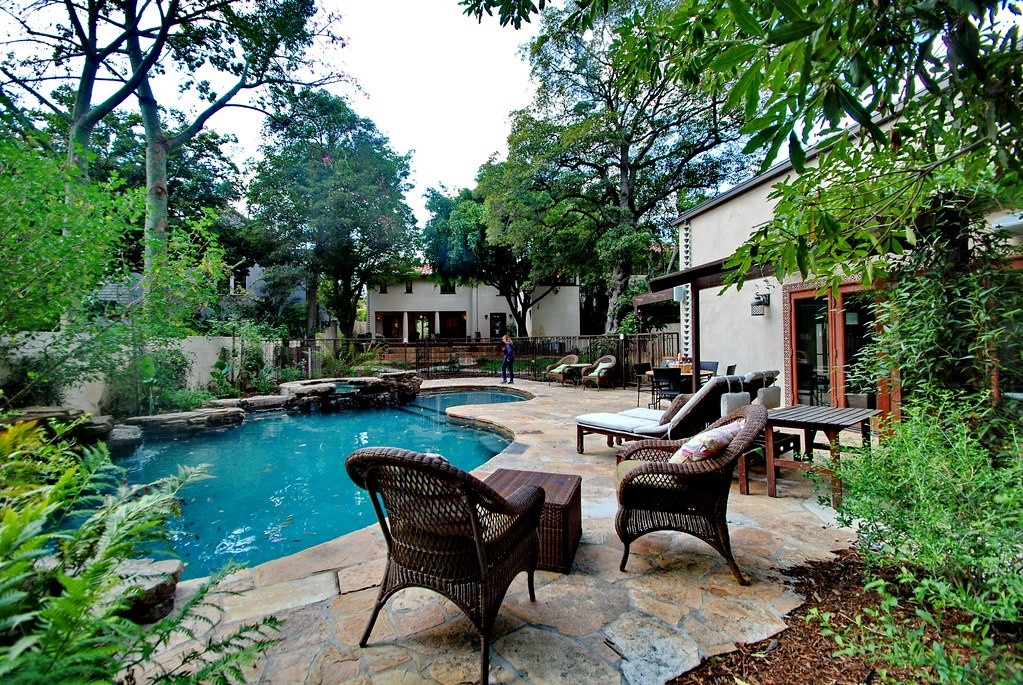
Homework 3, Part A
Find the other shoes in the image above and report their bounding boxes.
[508,380,514,384]
[501,379,507,383]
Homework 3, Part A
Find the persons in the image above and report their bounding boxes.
[500,335,514,384]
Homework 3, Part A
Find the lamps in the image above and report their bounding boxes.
[750,292,769,316]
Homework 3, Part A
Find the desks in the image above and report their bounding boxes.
[645,369,715,377]
[561,363,592,389]
[482,469,584,576]
[765,403,885,509]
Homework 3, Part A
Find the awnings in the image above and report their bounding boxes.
[649,191,972,292]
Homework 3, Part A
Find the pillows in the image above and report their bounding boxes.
[658,393,696,427]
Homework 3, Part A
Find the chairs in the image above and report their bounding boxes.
[581,354,616,392]
[344,446,546,685]
[618,369,781,423]
[634,363,655,407]
[700,361,719,390]
[546,354,579,388]
[616,404,768,588]
[574,375,746,454]
[660,357,678,368]
[652,367,681,410]
